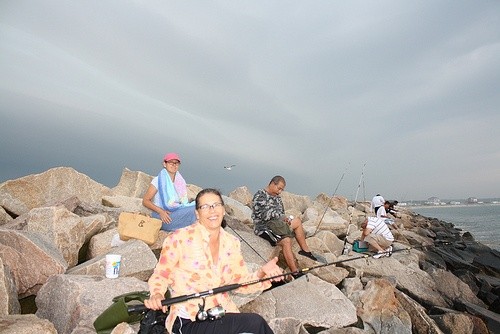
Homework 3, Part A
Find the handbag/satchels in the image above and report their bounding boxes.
[140,309,167,334]
[93,289,171,334]
[266,218,290,235]
[118,211,162,246]
[352,240,369,253]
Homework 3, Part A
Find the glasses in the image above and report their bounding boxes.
[197,203,223,210]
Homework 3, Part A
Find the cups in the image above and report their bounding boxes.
[288,215,294,226]
[105,254,121,280]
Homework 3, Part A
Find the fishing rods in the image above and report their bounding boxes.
[125,244,436,314]
[312,171,346,237]
[226,223,268,262]
[340,163,366,254]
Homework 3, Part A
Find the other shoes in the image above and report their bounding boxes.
[292,272,304,279]
[299,251,317,261]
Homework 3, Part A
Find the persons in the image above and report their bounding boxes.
[371,194,385,215]
[142,152,202,231]
[357,216,394,259]
[377,201,398,230]
[252,176,317,279]
[144,189,273,333]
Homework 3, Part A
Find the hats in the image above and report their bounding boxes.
[376,193,381,196]
[357,216,365,229]
[164,153,181,163]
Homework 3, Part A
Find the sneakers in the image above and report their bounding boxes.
[373,251,386,259]
[385,246,392,257]
